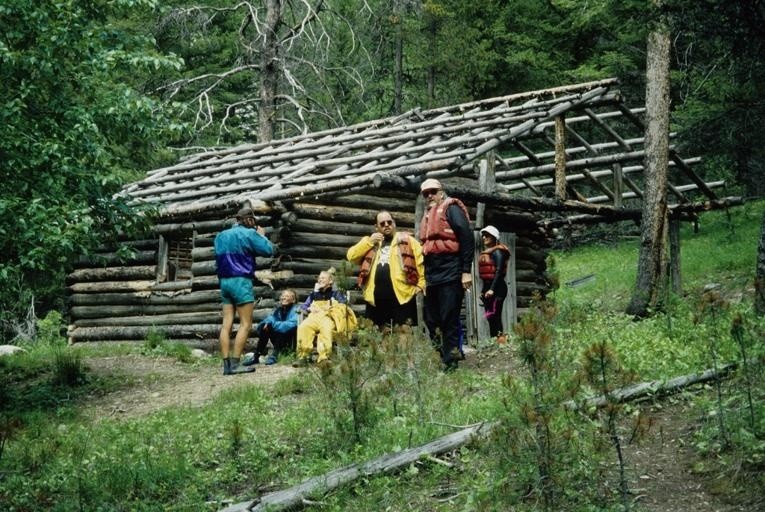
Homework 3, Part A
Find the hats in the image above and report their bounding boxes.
[480,225,500,240]
[238,209,257,219]
[421,179,441,191]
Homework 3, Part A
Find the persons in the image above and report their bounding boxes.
[242,290,303,366]
[477,225,512,340]
[290,270,348,368]
[213,208,275,374]
[346,209,426,331]
[418,178,476,374]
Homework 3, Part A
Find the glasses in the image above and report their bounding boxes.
[378,220,393,227]
[423,189,439,198]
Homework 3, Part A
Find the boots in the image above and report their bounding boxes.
[223,357,256,374]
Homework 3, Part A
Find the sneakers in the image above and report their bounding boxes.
[292,358,313,368]
[243,355,259,366]
[265,356,279,365]
